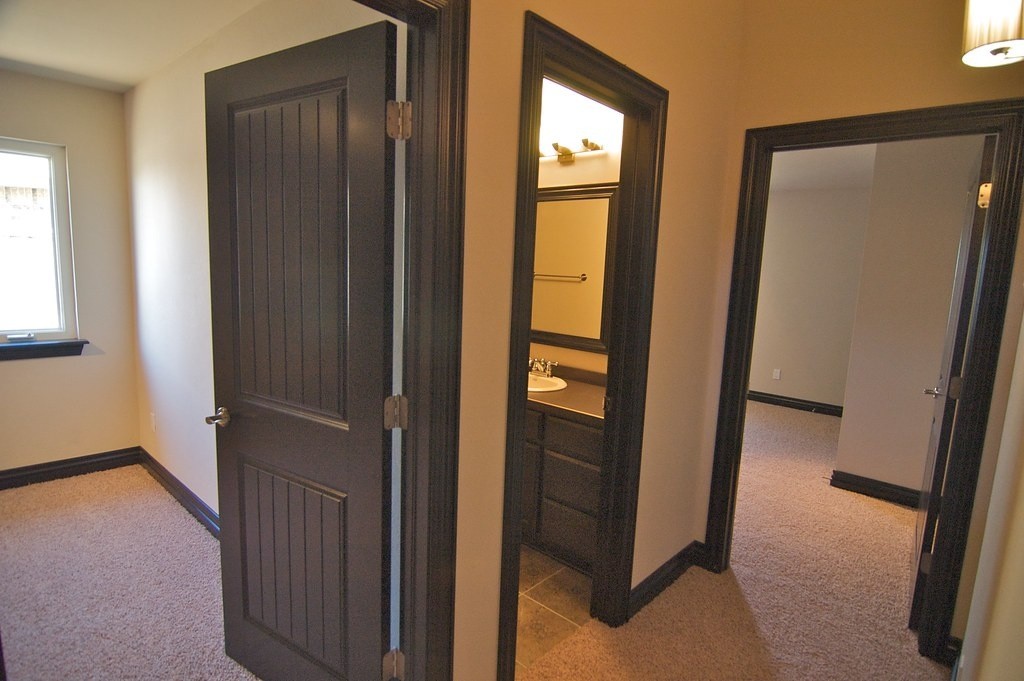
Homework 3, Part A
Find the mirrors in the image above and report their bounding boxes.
[531,182,620,355]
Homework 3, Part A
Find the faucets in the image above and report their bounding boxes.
[532,358,546,372]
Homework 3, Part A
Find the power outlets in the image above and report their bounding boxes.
[773,368,780,379]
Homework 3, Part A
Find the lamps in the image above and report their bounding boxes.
[961,0,1024,69]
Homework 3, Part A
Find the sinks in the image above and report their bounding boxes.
[529,375,568,392]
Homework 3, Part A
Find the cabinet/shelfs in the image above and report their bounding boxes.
[522,410,603,577]
